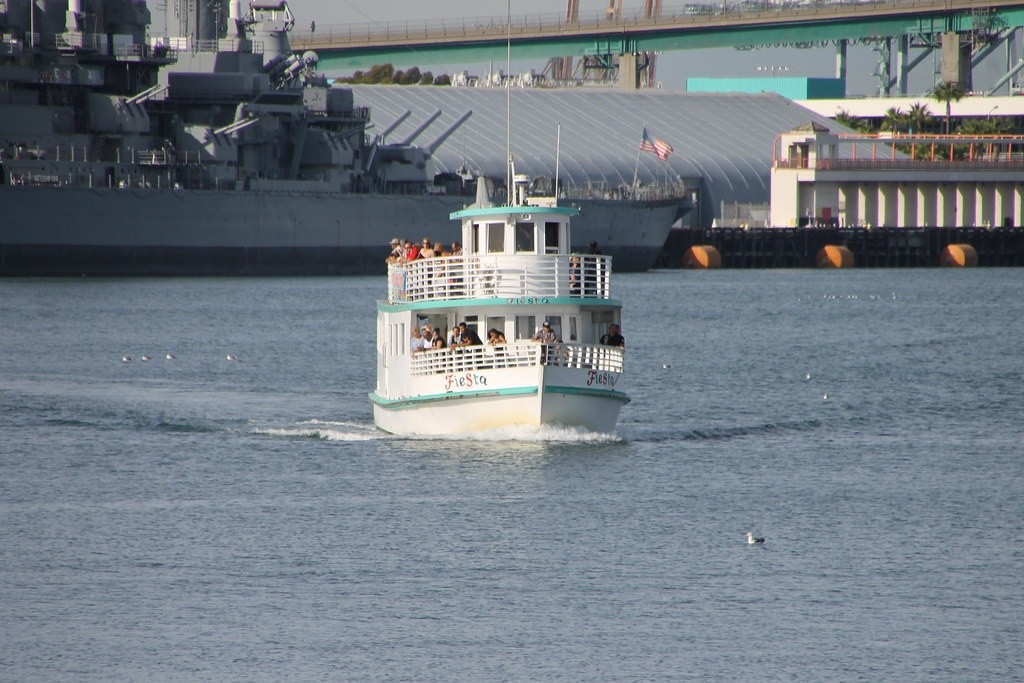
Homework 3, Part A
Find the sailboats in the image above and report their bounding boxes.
[370,1,631,440]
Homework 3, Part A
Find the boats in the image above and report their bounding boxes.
[662,120,1024,268]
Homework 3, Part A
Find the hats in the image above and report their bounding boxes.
[569,252,578,261]
[388,238,400,244]
[421,324,431,331]
[543,321,551,327]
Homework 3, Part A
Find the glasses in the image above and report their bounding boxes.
[422,242,430,244]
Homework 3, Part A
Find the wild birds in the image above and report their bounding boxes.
[660,290,898,400]
[744,532,768,545]
[121,353,177,362]
[226,354,237,361]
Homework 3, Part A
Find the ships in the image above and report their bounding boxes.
[0,0,695,277]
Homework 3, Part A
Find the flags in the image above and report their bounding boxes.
[640,128,672,161]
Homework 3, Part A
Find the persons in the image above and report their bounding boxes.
[531,321,569,367]
[385,238,463,298]
[569,241,604,298]
[487,329,510,368]
[599,323,625,371]
[410,321,484,375]
